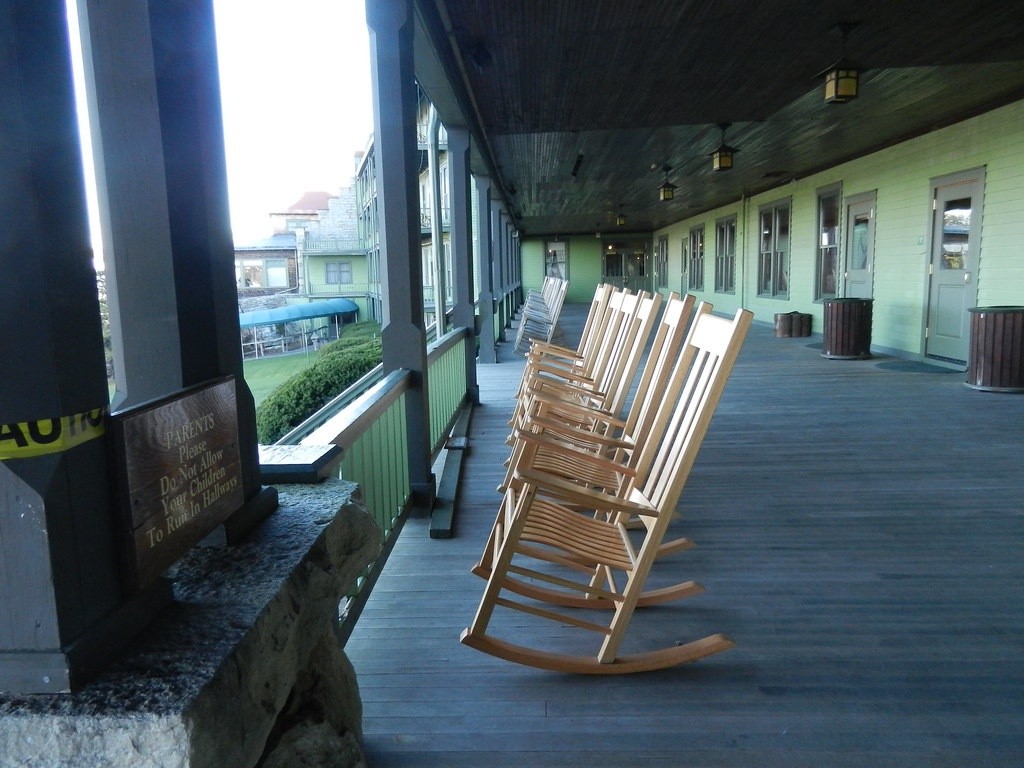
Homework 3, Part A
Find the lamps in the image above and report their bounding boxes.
[809,22,874,107]
[596,222,602,239]
[707,123,742,172]
[656,166,679,201]
[614,204,628,226]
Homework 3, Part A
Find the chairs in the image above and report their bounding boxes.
[456,274,756,677]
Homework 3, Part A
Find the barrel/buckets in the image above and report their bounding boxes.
[801,314,812,337]
[774,313,792,337]
[791,313,802,337]
[964,306,1024,393]
[820,298,875,359]
